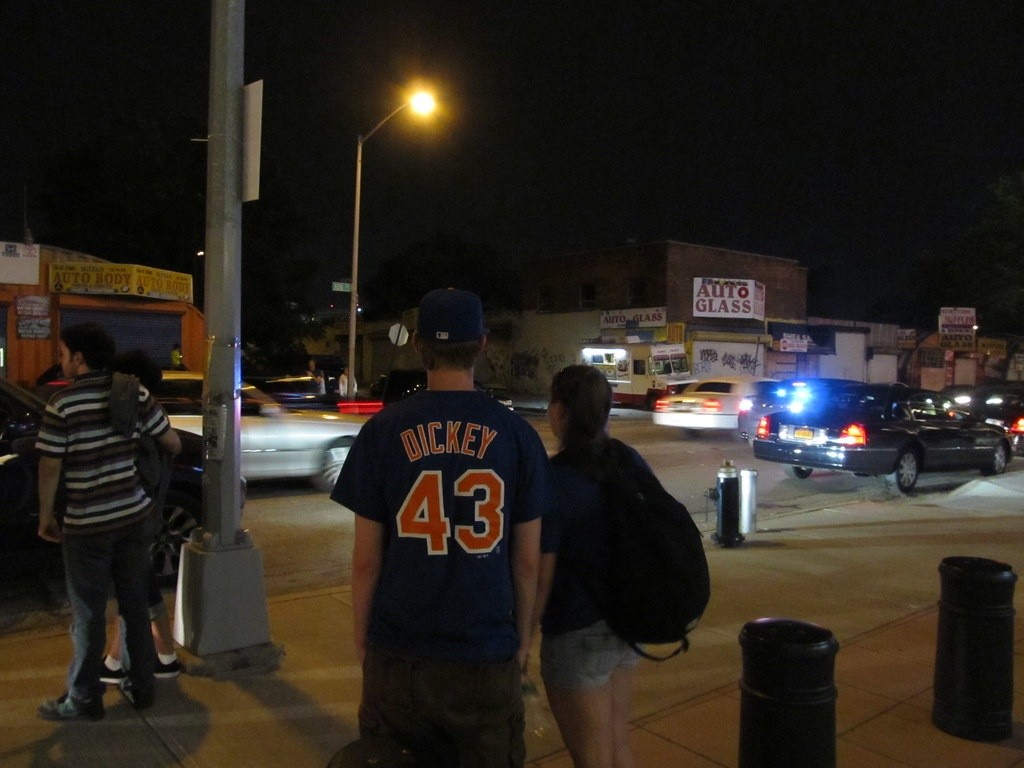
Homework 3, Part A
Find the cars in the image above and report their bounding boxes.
[651,377,782,435]
[159,371,363,493]
[737,376,864,439]
[941,384,1024,457]
[753,382,1013,495]
[0,379,249,590]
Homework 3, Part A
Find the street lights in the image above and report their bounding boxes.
[346,93,433,396]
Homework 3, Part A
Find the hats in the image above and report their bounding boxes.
[417,287,489,342]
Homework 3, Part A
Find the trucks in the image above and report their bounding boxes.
[578,341,700,410]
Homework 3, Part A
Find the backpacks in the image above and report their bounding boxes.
[553,438,711,662]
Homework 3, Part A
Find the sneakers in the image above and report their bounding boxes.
[152,653,179,679]
[37,689,105,722]
[98,656,122,684]
[117,678,154,707]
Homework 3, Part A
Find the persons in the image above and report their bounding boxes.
[100,348,180,683]
[523,363,656,768]
[339,365,358,397]
[35,320,182,720]
[330,287,560,768]
[306,358,323,384]
